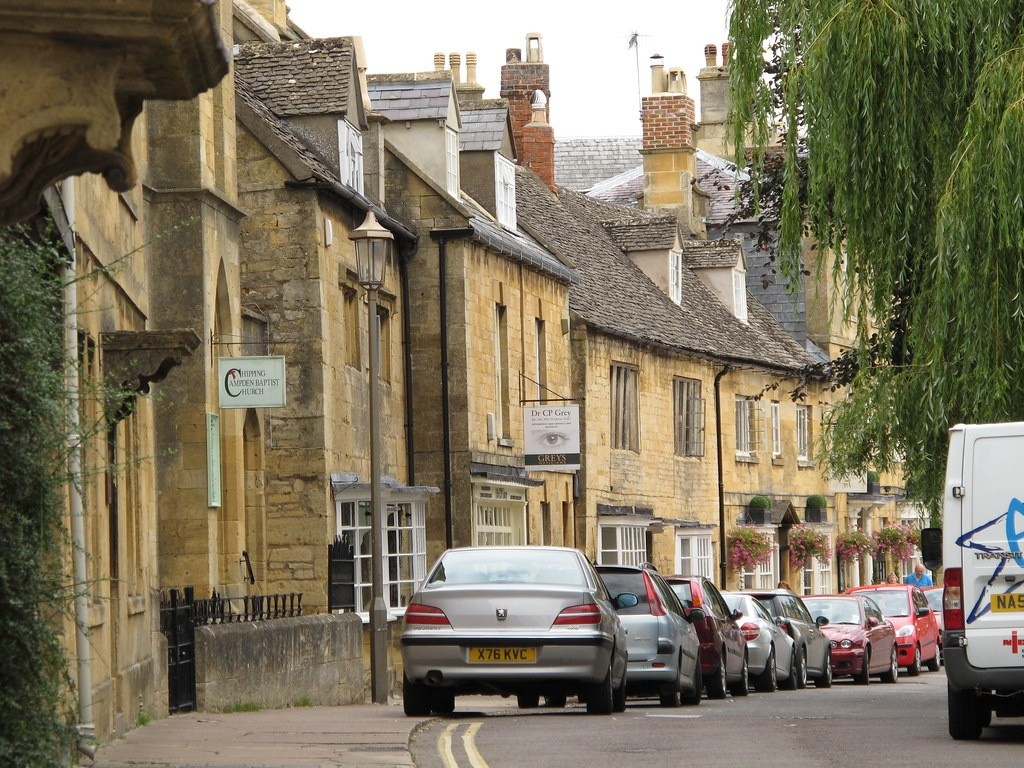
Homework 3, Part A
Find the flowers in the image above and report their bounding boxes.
[874,521,916,583]
[727,520,778,572]
[835,523,878,569]
[788,524,832,573]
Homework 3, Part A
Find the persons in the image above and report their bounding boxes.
[887,573,898,583]
[778,582,792,590]
[906,564,932,586]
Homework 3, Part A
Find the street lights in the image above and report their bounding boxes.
[711,364,734,600]
[346,202,393,708]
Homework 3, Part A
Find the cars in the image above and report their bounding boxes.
[720,594,798,694]
[799,593,900,684]
[921,587,945,635]
[660,573,748,701]
[396,545,639,721]
[588,556,705,713]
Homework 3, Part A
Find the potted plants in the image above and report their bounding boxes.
[746,496,771,524]
[804,494,827,524]
[867,470,880,494]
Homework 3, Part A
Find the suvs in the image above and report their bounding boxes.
[746,589,835,691]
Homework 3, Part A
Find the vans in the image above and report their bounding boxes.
[919,420,1024,739]
[842,584,942,678]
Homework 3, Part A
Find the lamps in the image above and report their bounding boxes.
[884,486,891,493]
